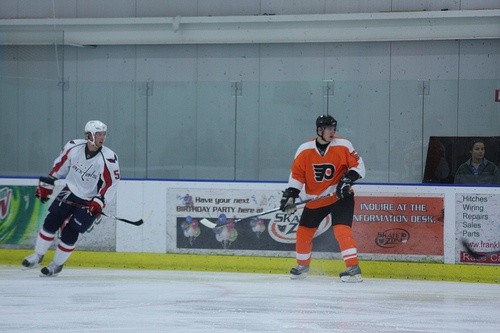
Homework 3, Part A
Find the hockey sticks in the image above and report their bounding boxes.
[200,188,354,229]
[38,194,144,227]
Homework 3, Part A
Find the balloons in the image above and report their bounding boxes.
[212,214,238,241]
[250,216,265,232]
[181,216,201,238]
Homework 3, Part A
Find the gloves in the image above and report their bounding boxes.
[335,170,362,199]
[33,177,55,204]
[278,187,300,214]
[86,196,105,216]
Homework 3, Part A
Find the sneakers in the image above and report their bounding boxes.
[21,253,44,267]
[41,261,63,277]
[338,265,364,283]
[288,264,310,280]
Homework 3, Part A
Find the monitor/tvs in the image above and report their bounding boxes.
[423,136,500,184]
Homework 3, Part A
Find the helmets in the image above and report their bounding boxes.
[316,114,337,127]
[84,120,108,134]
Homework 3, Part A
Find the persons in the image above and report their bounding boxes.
[454,140,500,183]
[22,119,120,277]
[280,114,365,282]
